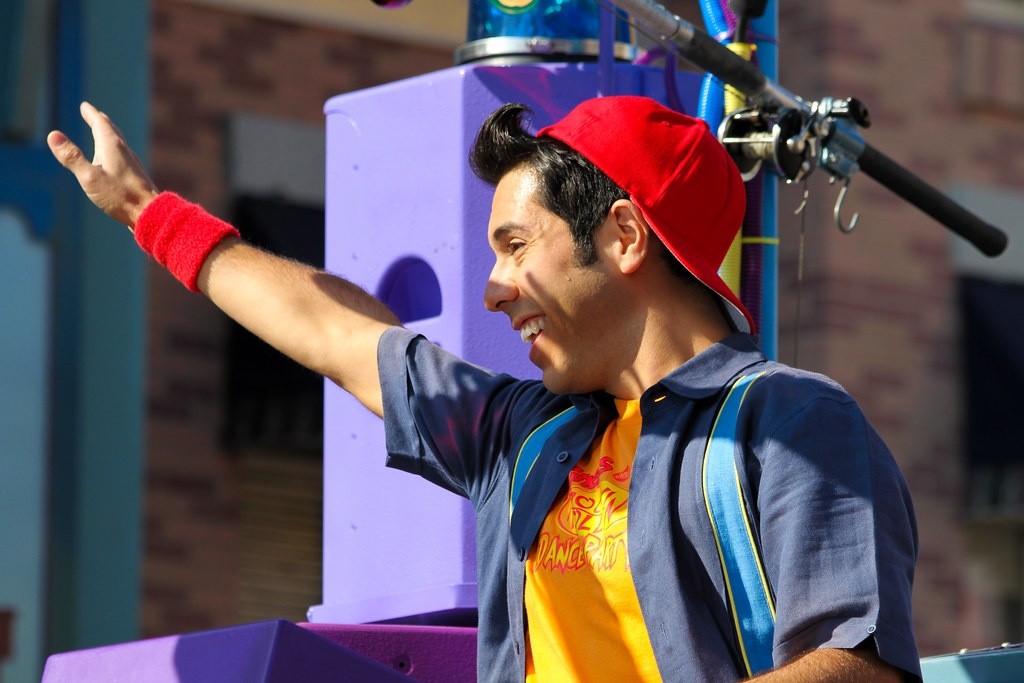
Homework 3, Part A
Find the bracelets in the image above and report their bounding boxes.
[134,190,241,295]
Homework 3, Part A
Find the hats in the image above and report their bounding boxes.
[535,96,756,338]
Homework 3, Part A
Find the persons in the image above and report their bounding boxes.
[46,94,923,683]
